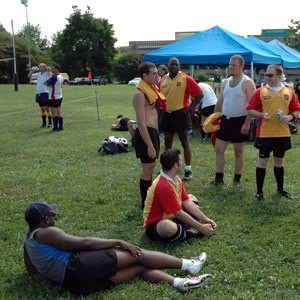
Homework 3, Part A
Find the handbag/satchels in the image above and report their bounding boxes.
[98,136,127,155]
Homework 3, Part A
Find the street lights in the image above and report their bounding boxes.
[20,0,32,68]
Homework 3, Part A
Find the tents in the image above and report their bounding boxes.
[144,25,300,80]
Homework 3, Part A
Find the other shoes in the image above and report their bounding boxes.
[254,193,264,202]
[48,124,52,127]
[186,227,204,237]
[52,128,58,131]
[40,125,46,127]
[58,127,63,130]
[186,130,193,138]
[212,181,224,186]
[277,191,292,199]
[229,181,240,188]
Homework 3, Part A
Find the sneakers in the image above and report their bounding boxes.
[178,274,213,293]
[187,252,207,275]
[184,169,193,179]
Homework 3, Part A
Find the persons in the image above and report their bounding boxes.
[213,69,221,94]
[142,148,217,243]
[194,78,218,142]
[27,63,64,132]
[111,114,130,131]
[132,62,161,210]
[280,67,300,102]
[24,201,215,292]
[245,64,300,202]
[158,57,204,180]
[206,54,257,188]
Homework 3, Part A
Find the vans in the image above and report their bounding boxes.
[57,72,70,84]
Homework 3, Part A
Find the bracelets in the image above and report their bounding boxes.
[290,113,296,119]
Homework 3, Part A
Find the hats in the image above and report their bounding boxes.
[25,200,58,225]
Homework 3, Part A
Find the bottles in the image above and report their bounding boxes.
[277,108,285,125]
[32,67,39,70]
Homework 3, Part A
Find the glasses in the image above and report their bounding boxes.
[265,74,279,78]
[49,213,55,217]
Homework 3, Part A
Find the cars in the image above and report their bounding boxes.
[128,77,141,86]
[29,73,37,85]
[68,76,91,85]
[91,74,110,86]
